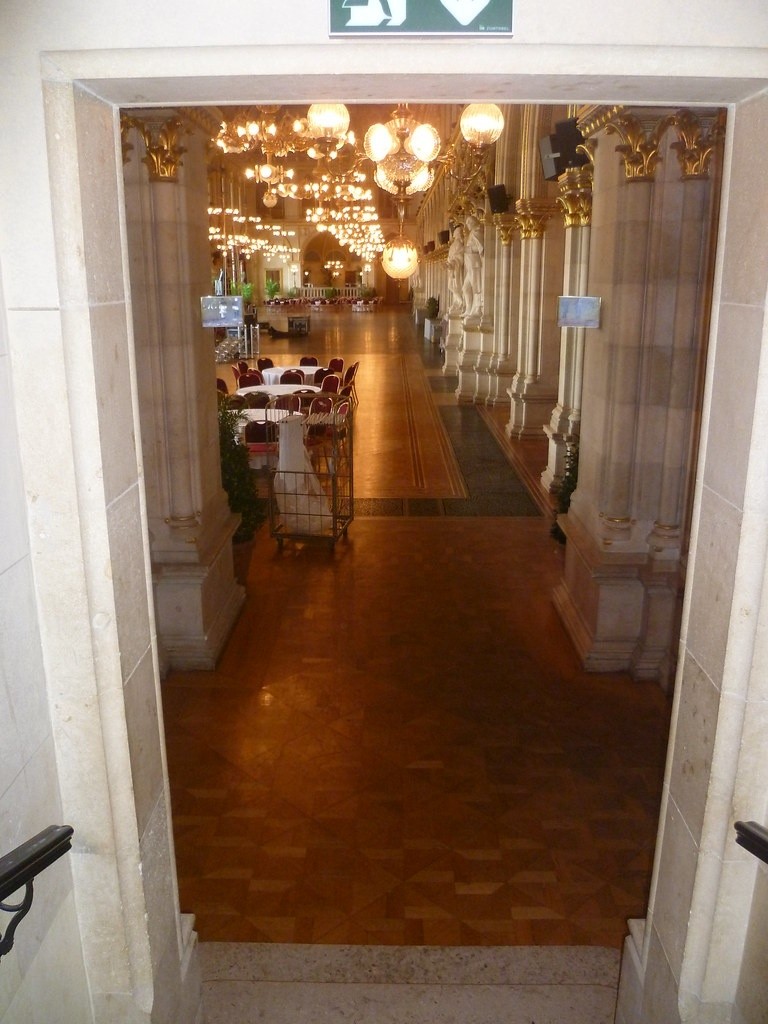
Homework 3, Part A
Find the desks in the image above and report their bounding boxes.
[351,300,373,312]
[279,302,284,305]
[262,366,324,386]
[219,409,304,433]
[269,302,274,305]
[236,384,321,409]
[315,301,321,306]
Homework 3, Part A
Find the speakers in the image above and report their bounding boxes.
[538,116,589,180]
[422,230,449,254]
[487,184,508,214]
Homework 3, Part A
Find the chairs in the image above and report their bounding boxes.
[265,297,384,312]
[216,355,360,476]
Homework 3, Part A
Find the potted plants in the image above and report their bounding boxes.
[423,297,442,340]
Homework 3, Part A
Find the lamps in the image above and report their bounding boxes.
[209,102,505,281]
